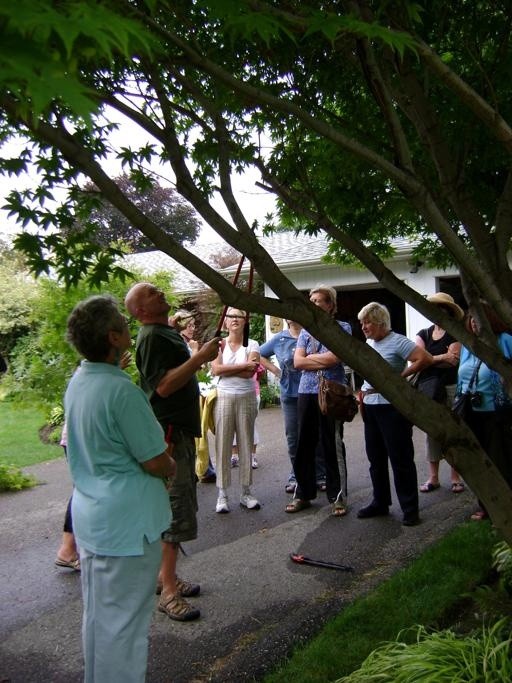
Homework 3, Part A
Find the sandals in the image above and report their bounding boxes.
[469,508,489,521]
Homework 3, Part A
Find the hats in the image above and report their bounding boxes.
[426,292,464,321]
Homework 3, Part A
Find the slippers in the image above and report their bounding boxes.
[452,482,464,493]
[285,499,311,512]
[421,482,440,492]
[55,552,81,570]
[331,502,347,515]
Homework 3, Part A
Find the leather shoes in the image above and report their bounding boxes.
[357,505,389,518]
[402,509,421,526]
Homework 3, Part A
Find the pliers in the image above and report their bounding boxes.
[214,254,254,347]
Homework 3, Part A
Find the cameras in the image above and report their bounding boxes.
[471,392,481,407]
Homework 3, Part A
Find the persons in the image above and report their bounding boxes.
[283,285,352,516]
[454,293,512,521]
[169,309,217,483]
[259,319,327,493]
[63,296,177,683]
[125,282,221,621]
[210,306,265,513]
[411,291,466,494]
[55,351,134,570]
[357,301,434,527]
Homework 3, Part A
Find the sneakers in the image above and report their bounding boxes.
[215,497,229,513]
[159,592,200,621]
[156,576,200,597]
[285,479,327,493]
[239,495,260,509]
[231,453,259,469]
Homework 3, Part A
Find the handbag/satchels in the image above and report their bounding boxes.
[318,376,358,422]
[451,392,470,422]
